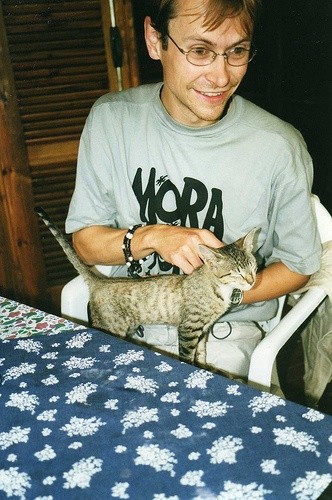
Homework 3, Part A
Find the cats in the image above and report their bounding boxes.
[35,202,262,370]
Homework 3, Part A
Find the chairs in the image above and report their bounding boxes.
[60,193,332,392]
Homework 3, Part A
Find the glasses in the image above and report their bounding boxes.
[165,28,257,66]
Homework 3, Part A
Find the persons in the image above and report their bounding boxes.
[60,1,324,384]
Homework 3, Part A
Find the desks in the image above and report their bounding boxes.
[0,297,332,500]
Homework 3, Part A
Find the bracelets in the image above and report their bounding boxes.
[122,224,145,267]
